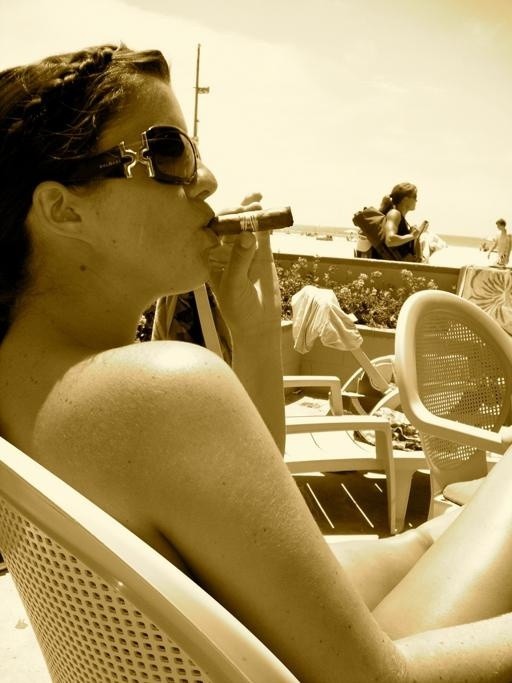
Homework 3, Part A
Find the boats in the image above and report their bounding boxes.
[308,231,333,241]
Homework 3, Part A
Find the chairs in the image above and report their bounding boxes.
[387,288,512,500]
[188,282,392,539]
[0,423,305,676]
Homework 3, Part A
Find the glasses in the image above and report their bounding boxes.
[31,125,204,187]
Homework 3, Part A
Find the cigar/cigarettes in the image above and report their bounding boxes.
[207,206,294,235]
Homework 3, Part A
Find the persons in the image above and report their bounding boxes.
[0,43,511,683]
[380,183,418,259]
[488,220,512,266]
